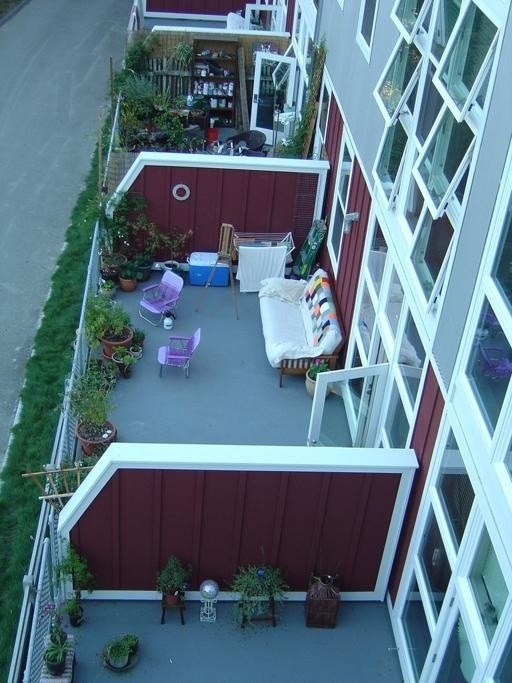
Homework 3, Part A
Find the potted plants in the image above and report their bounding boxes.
[305,363,332,398]
[106,634,139,668]
[44,545,97,676]
[81,191,194,299]
[121,71,188,134]
[66,292,145,456]
[156,555,192,605]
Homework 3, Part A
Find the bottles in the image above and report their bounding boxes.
[188,80,234,96]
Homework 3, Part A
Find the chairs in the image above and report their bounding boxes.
[139,270,184,327]
[227,130,266,152]
[479,346,512,382]
[158,327,201,378]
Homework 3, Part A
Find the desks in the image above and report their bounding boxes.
[39,635,76,682]
[161,596,186,625]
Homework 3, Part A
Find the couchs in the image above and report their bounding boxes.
[260,268,348,389]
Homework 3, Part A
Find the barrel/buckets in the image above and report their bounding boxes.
[306,574,340,630]
[206,128,218,147]
[163,260,179,272]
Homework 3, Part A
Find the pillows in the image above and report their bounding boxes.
[268,341,324,365]
[258,277,306,307]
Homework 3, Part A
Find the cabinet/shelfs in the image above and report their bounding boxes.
[190,37,239,129]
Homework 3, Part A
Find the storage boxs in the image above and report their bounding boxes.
[186,252,230,287]
